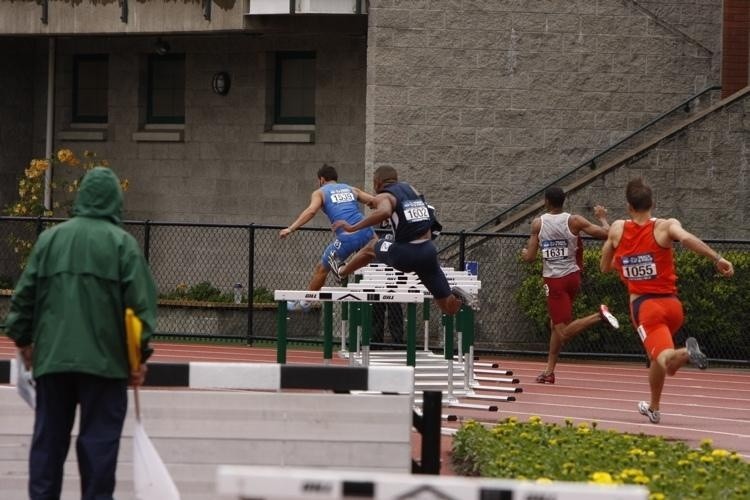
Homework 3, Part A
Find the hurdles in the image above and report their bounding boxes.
[0,358,445,472]
[216,463,650,500]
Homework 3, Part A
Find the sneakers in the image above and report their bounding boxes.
[599,304,620,331]
[287,301,311,312]
[451,285,481,312]
[534,371,555,385]
[685,336,709,370]
[327,249,348,284]
[638,399,662,425]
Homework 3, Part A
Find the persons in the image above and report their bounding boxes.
[600,178,733,425]
[1,165,158,500]
[327,166,472,316]
[279,163,378,310]
[522,186,620,384]
